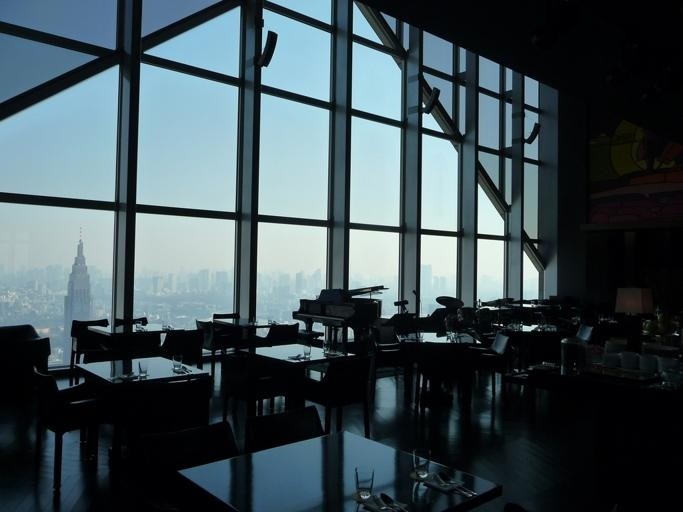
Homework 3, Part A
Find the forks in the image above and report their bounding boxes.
[432,472,472,497]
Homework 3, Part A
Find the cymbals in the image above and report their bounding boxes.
[437,296,461,308]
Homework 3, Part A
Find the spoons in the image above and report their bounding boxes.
[371,494,394,510]
[380,492,408,511]
[437,470,478,496]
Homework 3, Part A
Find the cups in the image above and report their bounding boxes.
[171,354,182,370]
[303,343,311,357]
[161,320,167,330]
[322,340,330,352]
[411,481,430,505]
[136,359,148,377]
[134,320,142,331]
[412,448,431,478]
[353,465,373,498]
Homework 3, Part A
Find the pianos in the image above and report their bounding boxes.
[292,285,384,353]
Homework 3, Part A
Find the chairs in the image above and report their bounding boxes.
[0,294,681,510]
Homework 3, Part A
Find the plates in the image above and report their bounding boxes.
[289,354,304,359]
